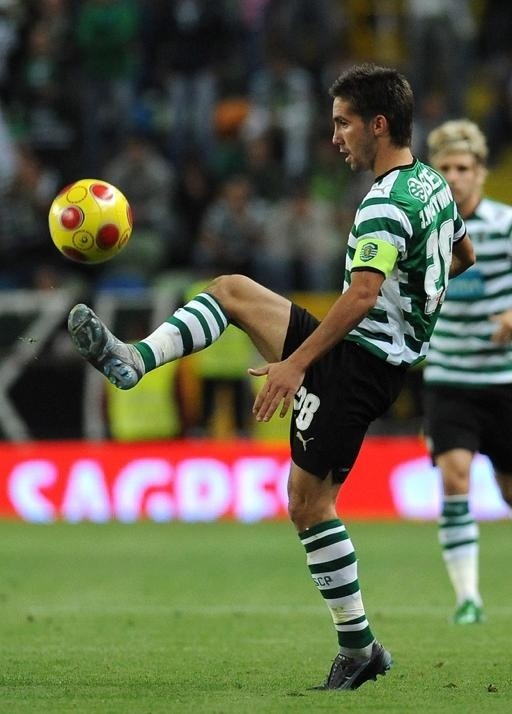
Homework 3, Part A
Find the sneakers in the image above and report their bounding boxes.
[308,638,395,695]
[449,593,485,627]
[66,299,148,391]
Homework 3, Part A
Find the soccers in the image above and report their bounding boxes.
[48,178,134,265]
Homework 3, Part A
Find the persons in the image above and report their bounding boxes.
[417,118,511,631]
[68,63,477,697]
[0,1,512,440]
